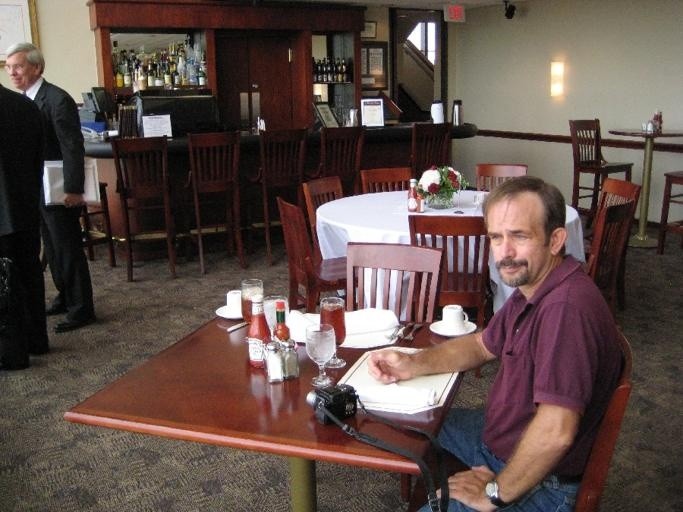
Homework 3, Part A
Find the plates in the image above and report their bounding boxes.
[428,320,477,337]
[214,305,244,320]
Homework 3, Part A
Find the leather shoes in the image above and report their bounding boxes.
[45,300,67,313]
[52,312,98,332]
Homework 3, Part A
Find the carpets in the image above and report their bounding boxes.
[0,217,681,512]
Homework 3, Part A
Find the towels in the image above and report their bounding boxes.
[283,308,401,350]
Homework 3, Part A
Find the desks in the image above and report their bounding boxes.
[608,129,683,248]
[315,191,586,320]
[64,312,483,512]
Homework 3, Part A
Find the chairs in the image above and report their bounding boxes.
[347,242,445,322]
[582,199,638,309]
[361,167,416,193]
[250,127,309,266]
[409,215,492,378]
[657,170,683,254]
[276,197,358,312]
[412,123,452,180]
[476,163,528,191]
[109,134,178,283]
[185,130,249,275]
[297,126,367,206]
[41,181,116,273]
[584,177,643,260]
[407,324,634,512]
[302,176,343,259]
[569,118,634,230]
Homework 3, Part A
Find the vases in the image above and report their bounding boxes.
[427,194,455,209]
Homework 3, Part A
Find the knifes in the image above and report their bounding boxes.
[389,322,406,341]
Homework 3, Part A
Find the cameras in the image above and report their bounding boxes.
[306,384,359,425]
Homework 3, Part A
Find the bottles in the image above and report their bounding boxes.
[172,64,180,86]
[647,121,653,132]
[115,65,124,88]
[136,49,172,88]
[247,294,271,368]
[658,112,662,133]
[336,57,342,82]
[653,113,658,132]
[408,179,417,212]
[266,343,284,383]
[317,60,323,82]
[273,302,290,341]
[417,184,425,213]
[313,57,317,83]
[124,64,131,87]
[322,60,327,82]
[642,123,646,131]
[198,68,206,88]
[282,340,299,380]
[110,39,136,87]
[327,57,334,82]
[147,62,155,87]
[342,58,347,82]
[331,57,337,82]
[171,37,207,86]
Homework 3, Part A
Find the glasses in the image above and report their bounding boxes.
[4,63,36,70]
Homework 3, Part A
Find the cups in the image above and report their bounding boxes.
[431,99,445,123]
[451,99,464,126]
[442,304,469,332]
[227,290,242,314]
[306,323,336,388]
[241,279,264,329]
[453,167,464,214]
[319,296,347,369]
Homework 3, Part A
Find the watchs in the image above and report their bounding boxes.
[485,478,511,507]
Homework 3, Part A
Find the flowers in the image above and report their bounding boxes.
[417,165,467,205]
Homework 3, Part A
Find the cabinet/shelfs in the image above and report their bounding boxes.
[85,0,366,126]
[84,124,477,262]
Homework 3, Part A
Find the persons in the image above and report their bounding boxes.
[368,176,627,512]
[1,83,51,370]
[6,42,95,332]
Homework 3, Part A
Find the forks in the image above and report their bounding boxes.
[396,323,415,343]
[402,323,424,344]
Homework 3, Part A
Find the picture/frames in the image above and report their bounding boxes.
[311,102,341,127]
[361,41,388,91]
[360,98,385,126]
[141,114,173,138]
[0,0,41,69]
[361,20,378,39]
